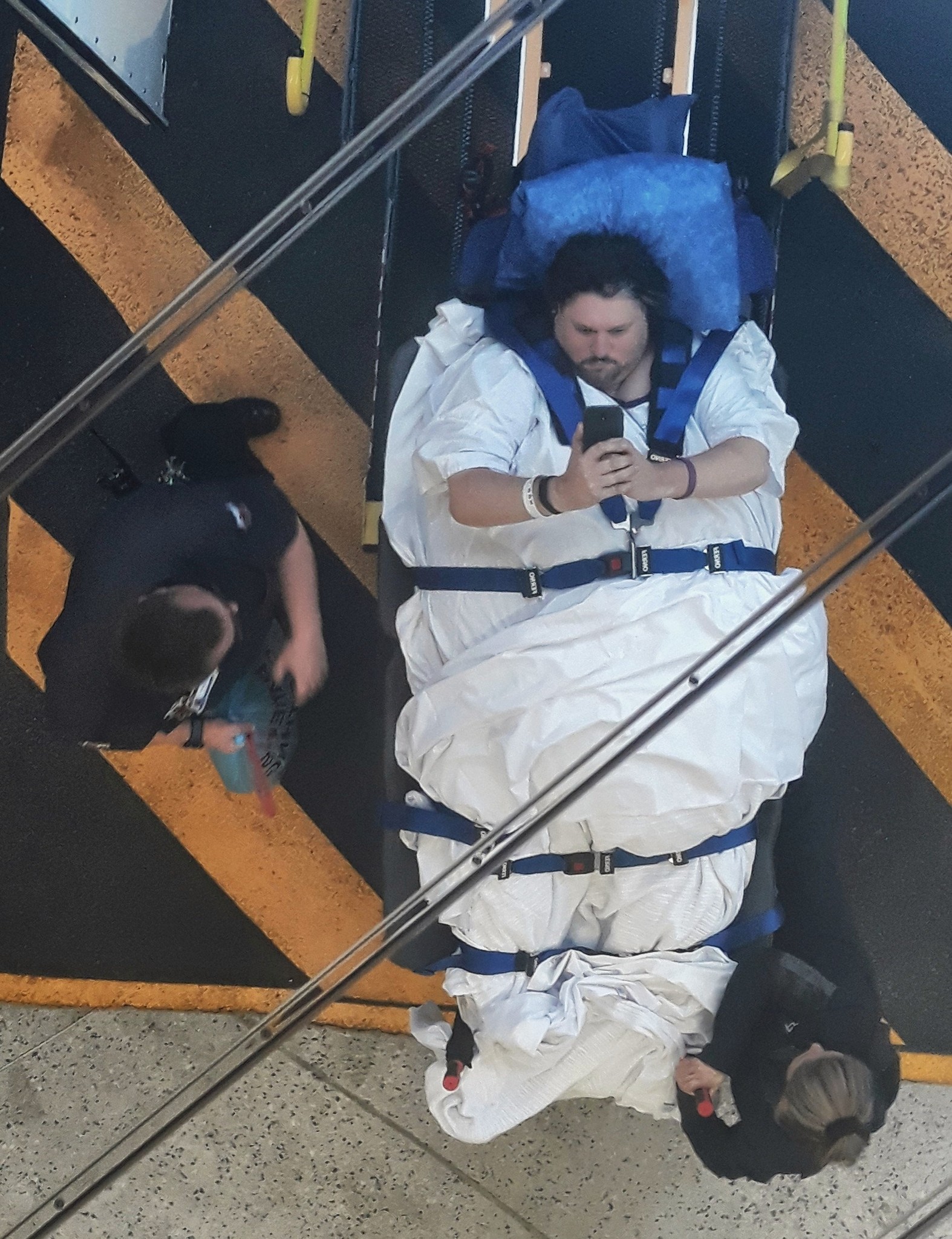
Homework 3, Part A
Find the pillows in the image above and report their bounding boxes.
[490,84,697,340]
[503,150,741,333]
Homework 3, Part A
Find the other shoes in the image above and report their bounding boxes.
[223,397,280,434]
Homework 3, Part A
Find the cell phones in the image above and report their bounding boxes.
[583,405,624,453]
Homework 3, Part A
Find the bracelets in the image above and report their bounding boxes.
[181,714,206,749]
[523,474,553,518]
[539,475,564,514]
[34,397,329,753]
[673,457,697,500]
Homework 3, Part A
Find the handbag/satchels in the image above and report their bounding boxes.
[201,673,300,816]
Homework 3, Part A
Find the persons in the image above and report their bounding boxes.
[384,222,830,1143]
[674,805,899,1187]
